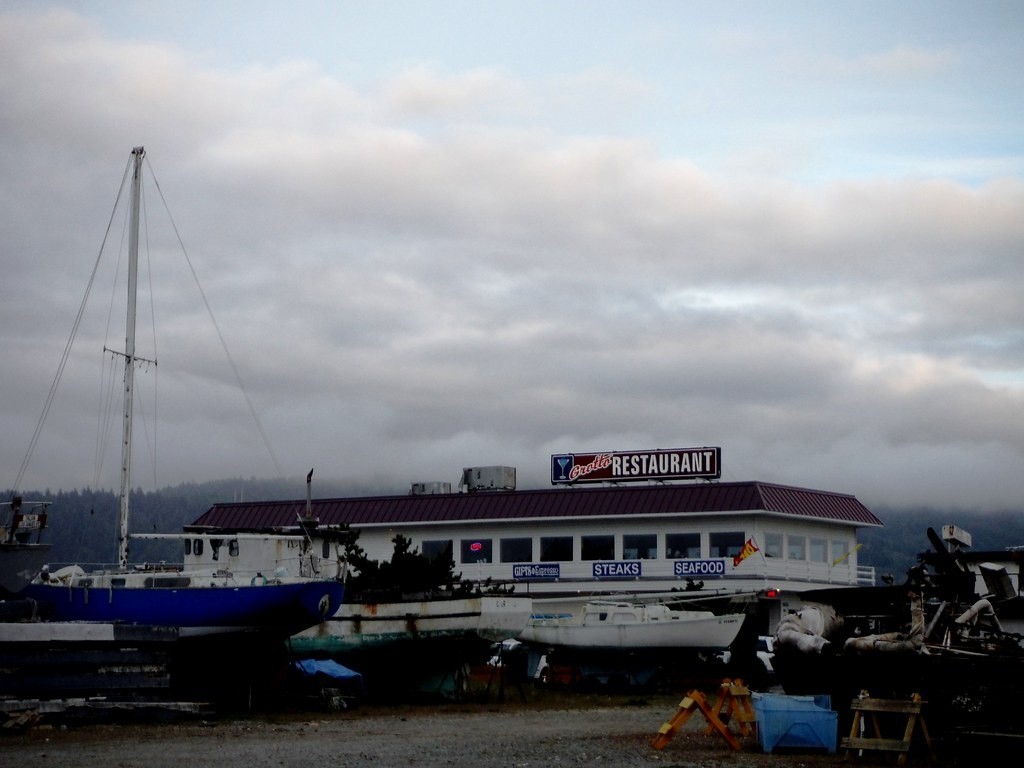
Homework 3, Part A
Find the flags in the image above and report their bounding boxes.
[733,539,759,566]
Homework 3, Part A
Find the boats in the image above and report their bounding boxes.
[1,144,748,700]
[772,525,1024,737]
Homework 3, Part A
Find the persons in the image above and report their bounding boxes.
[39,561,50,584]
[904,552,936,639]
[250,570,267,585]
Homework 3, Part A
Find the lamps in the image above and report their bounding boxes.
[881,574,895,586]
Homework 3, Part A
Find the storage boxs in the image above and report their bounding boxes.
[751,692,838,754]
